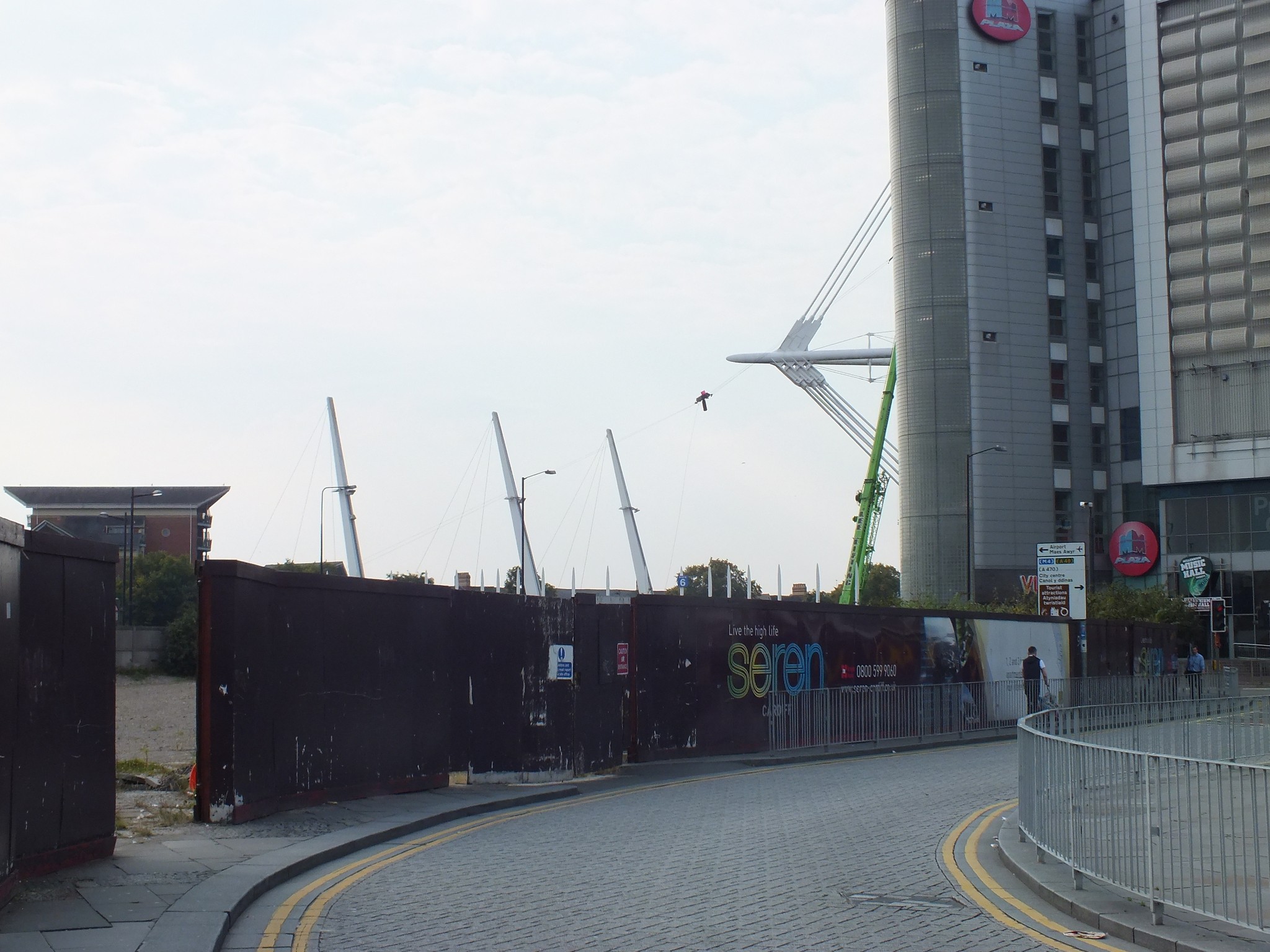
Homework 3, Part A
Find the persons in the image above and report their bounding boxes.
[1020,646,1050,714]
[1183,645,1205,704]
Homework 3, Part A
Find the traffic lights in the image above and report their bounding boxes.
[1210,599,1227,633]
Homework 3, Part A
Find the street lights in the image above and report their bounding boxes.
[320,485,357,574]
[966,444,1009,601]
[129,487,163,625]
[99,512,127,625]
[520,469,557,595]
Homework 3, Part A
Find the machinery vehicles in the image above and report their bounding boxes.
[837,340,897,603]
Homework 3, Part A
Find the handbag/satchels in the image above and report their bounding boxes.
[1184,669,1189,678]
